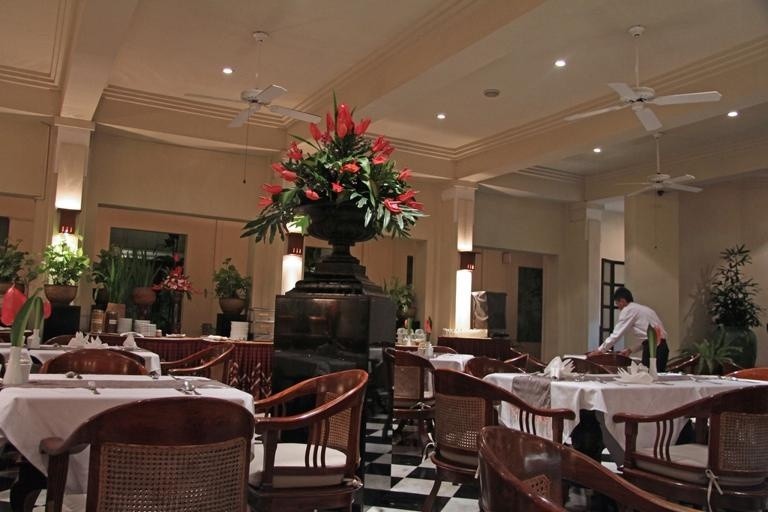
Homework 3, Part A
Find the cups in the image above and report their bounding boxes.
[156,329,161,337]
[418,341,434,358]
[574,369,586,382]
[442,327,489,339]
[396,328,424,346]
[208,335,227,340]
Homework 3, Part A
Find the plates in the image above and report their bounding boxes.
[614,378,654,384]
[116,317,157,335]
[690,375,726,382]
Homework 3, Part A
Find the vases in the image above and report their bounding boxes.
[43,284,78,306]
[395,308,416,319]
[218,298,245,316]
[169,294,181,303]
[303,202,376,246]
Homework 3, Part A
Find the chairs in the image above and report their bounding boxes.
[1,342,768,512]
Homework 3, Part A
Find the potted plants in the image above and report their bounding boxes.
[1,239,38,294]
[96,253,131,318]
[128,248,159,306]
[704,245,764,377]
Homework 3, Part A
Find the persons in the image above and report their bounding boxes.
[586,287,669,373]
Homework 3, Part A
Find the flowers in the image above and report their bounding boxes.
[157,266,205,301]
[646,322,661,358]
[212,257,251,300]
[0,283,51,347]
[240,88,431,245]
[389,283,414,312]
[37,242,90,286]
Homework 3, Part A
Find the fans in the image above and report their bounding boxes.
[183,32,322,129]
[562,26,722,132]
[616,139,704,196]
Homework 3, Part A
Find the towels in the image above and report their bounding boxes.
[119,331,144,350]
[167,333,186,338]
[68,331,107,346]
[203,334,230,342]
